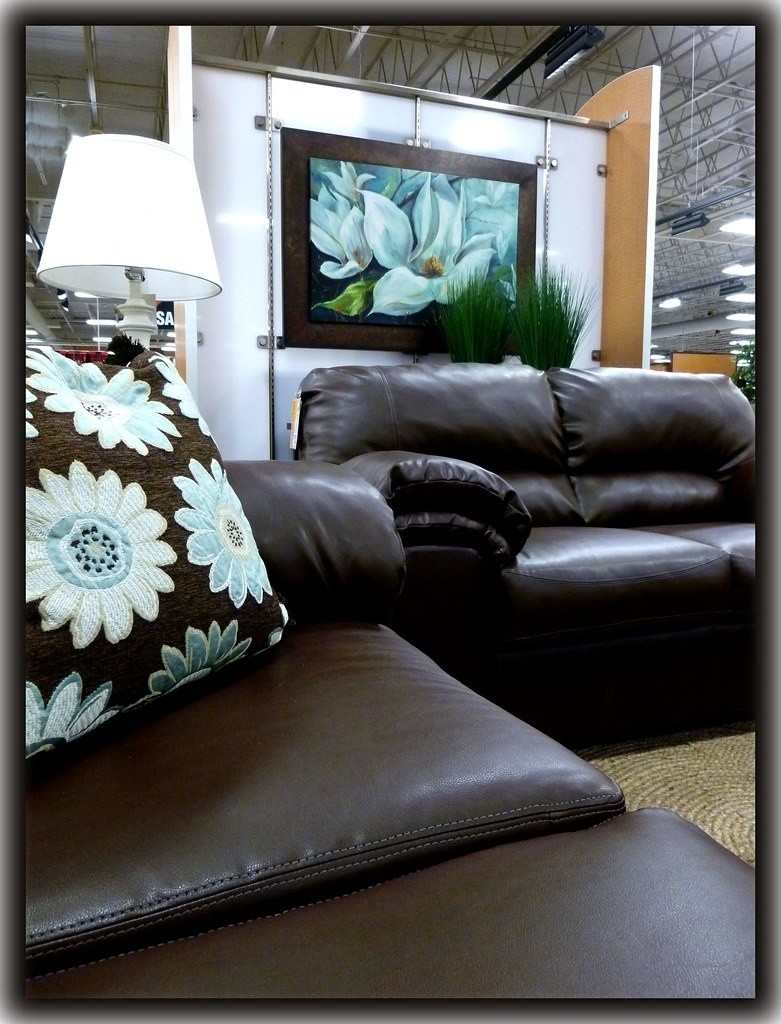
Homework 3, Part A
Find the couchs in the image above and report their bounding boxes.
[294,363,756,745]
[26,460,755,999]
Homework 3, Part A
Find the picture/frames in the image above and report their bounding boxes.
[279,126,537,358]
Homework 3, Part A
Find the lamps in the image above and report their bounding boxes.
[543,26,605,79]
[35,133,222,350]
[670,212,709,236]
[719,280,747,296]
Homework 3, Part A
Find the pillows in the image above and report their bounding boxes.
[25,341,288,760]
